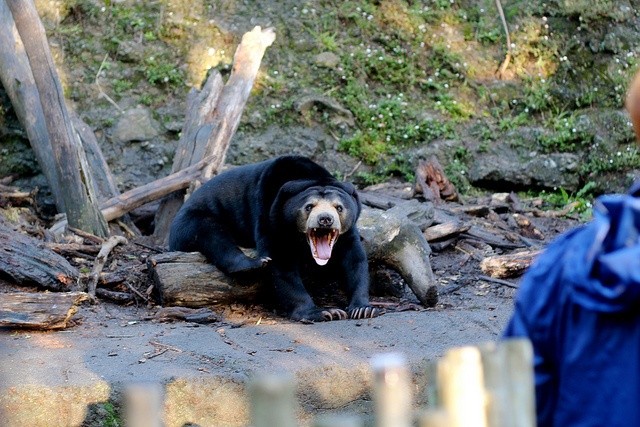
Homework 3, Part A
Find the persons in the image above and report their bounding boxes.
[497,71,640,427]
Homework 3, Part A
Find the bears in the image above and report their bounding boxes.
[167,154,384,324]
